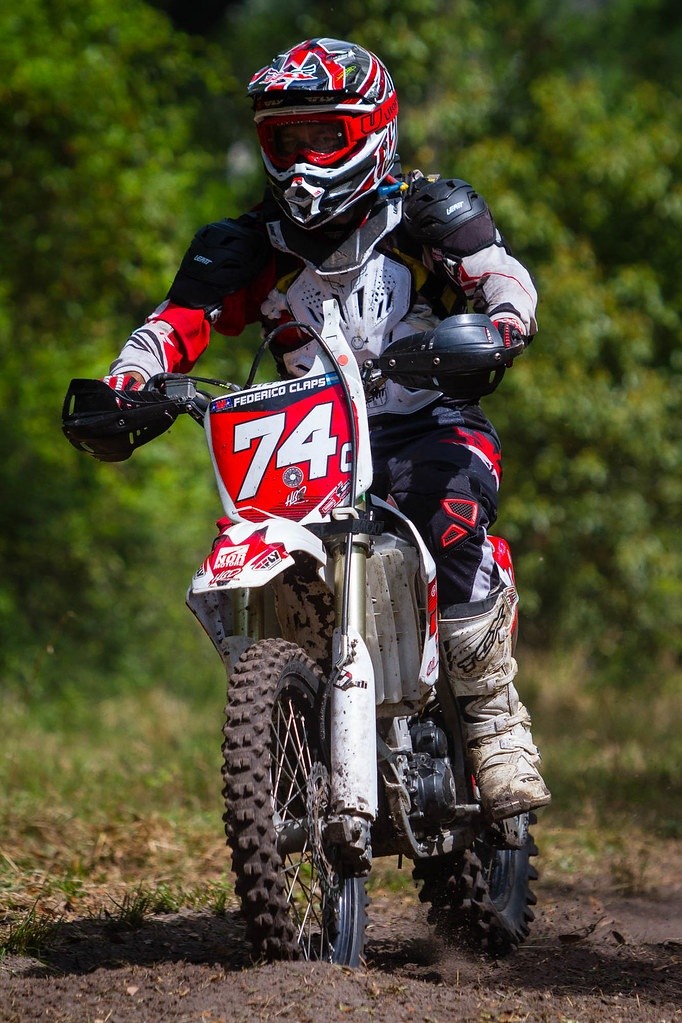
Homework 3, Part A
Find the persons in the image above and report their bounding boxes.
[104,34,553,820]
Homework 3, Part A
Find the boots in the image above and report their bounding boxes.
[438,589,550,818]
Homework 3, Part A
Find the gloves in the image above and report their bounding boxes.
[489,316,524,368]
[94,374,144,412]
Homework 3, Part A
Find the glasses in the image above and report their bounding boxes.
[258,113,355,170]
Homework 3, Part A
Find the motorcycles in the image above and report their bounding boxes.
[61,310,540,965]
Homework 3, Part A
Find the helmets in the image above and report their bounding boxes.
[247,37,399,231]
